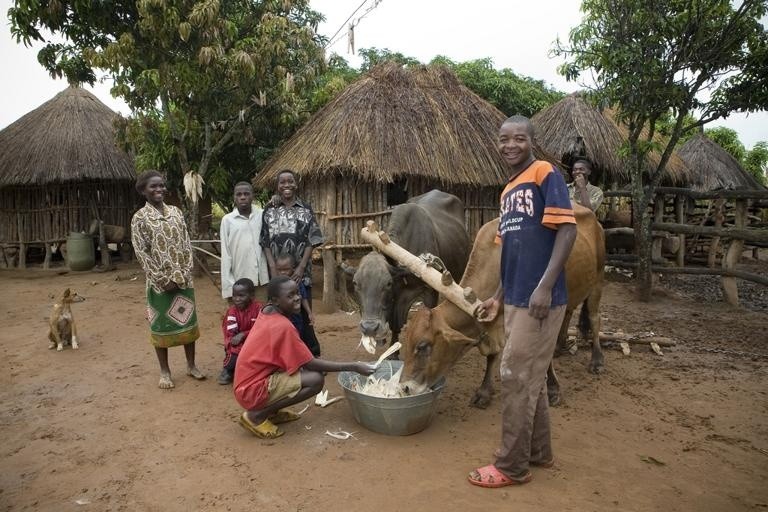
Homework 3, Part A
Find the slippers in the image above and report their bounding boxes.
[468,463,531,487]
[238,407,301,439]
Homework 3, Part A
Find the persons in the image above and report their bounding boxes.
[216,278,266,385]
[259,168,325,357]
[467,116,577,487]
[233,275,376,439]
[566,159,604,218]
[220,182,271,310]
[131,170,207,390]
[275,255,316,338]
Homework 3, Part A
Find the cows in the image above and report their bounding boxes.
[604,209,632,254]
[337,190,470,360]
[397,200,606,410]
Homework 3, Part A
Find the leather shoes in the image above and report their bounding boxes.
[218,368,232,384]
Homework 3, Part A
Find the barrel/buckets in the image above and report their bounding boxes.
[65,237,96,272]
[338,359,447,436]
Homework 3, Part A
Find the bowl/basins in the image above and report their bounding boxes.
[337,358,449,437]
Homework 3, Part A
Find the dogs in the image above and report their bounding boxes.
[47,288,86,352]
